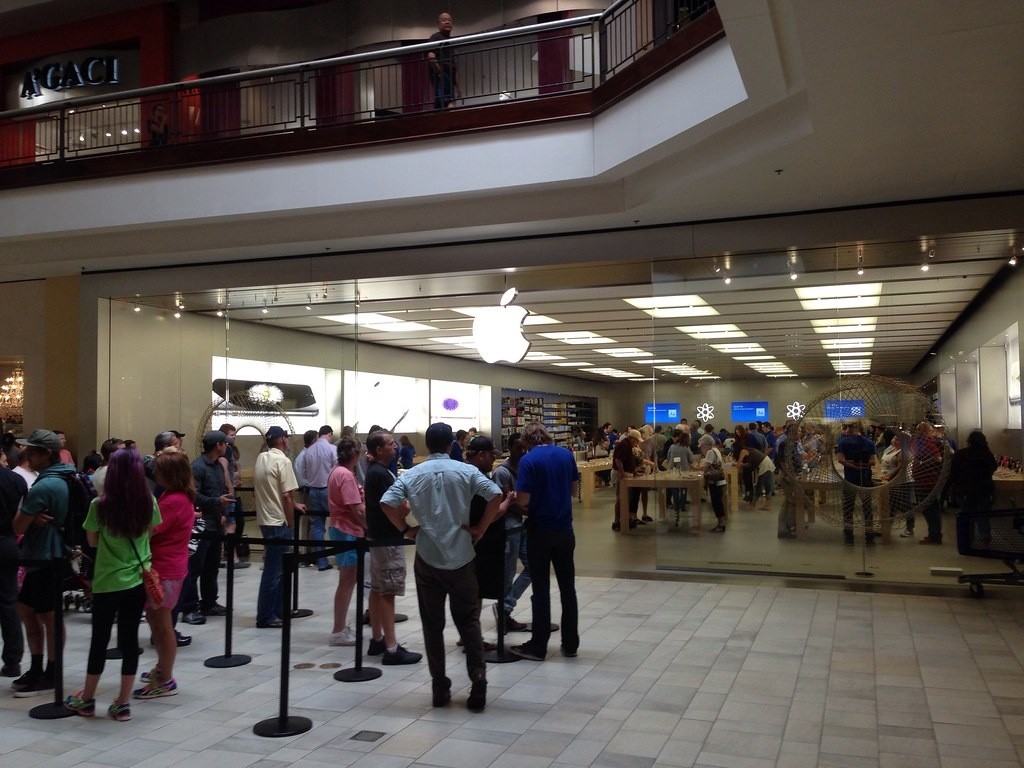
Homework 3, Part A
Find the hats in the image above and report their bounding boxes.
[628,430,643,442]
[467,435,502,456]
[15,429,61,452]
[168,430,185,437]
[202,430,233,446]
[266,426,292,440]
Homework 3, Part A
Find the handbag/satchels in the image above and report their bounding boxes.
[703,449,725,481]
[596,445,608,456]
[143,568,164,611]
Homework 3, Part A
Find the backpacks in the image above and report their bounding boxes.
[60,469,98,545]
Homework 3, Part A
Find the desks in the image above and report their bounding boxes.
[795,454,892,545]
[619,470,706,534]
[992,468,1024,509]
[722,456,746,511]
[576,458,613,507]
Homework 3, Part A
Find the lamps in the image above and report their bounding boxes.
[712,246,1019,284]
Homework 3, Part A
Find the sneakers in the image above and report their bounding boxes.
[330,623,363,645]
[381,642,422,665]
[493,603,528,634]
[134,678,178,698]
[509,642,546,660]
[64,691,95,715]
[901,529,915,536]
[182,613,206,623]
[141,672,160,682]
[368,635,385,655]
[201,605,233,616]
[562,646,577,656]
[108,697,131,721]
[11,669,55,697]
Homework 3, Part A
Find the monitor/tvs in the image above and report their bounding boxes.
[825,400,865,419]
[646,403,680,423]
[731,401,768,423]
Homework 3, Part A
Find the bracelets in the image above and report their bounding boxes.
[400,526,411,536]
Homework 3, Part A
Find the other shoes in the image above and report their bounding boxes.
[0,663,21,676]
[918,536,939,544]
[256,618,284,627]
[844,540,853,545]
[466,687,486,707]
[865,541,874,547]
[433,690,451,706]
[612,515,653,531]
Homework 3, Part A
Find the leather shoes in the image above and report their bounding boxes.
[174,630,192,645]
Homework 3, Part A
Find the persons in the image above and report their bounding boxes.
[147,104,169,147]
[132,452,197,699]
[293,425,416,571]
[775,419,834,539]
[717,421,775,510]
[379,423,503,713]
[0,430,77,698]
[425,11,462,109]
[834,421,999,551]
[142,424,251,647]
[254,426,308,628]
[327,436,369,646]
[64,447,163,721]
[572,423,622,488]
[82,438,137,623]
[450,421,579,662]
[610,419,728,533]
[364,430,423,666]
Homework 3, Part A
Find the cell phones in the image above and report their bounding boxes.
[211,379,318,417]
